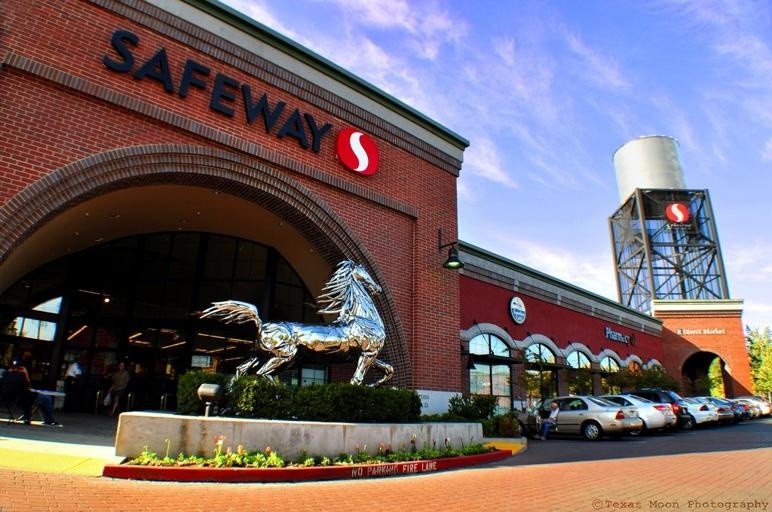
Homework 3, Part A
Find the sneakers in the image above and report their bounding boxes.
[534,434,546,440]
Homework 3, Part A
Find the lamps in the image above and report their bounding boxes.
[461,352,476,369]
[440,241,464,269]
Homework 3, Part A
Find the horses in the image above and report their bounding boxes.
[198,256,397,399]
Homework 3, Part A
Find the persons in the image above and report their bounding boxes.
[62,356,83,404]
[533,401,560,441]
[0,358,21,419]
[5,357,66,430]
[106,362,130,419]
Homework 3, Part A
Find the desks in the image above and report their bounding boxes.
[17,388,66,421]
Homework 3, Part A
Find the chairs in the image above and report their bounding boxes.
[0,380,39,424]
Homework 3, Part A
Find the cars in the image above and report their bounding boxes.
[530,388,772,440]
